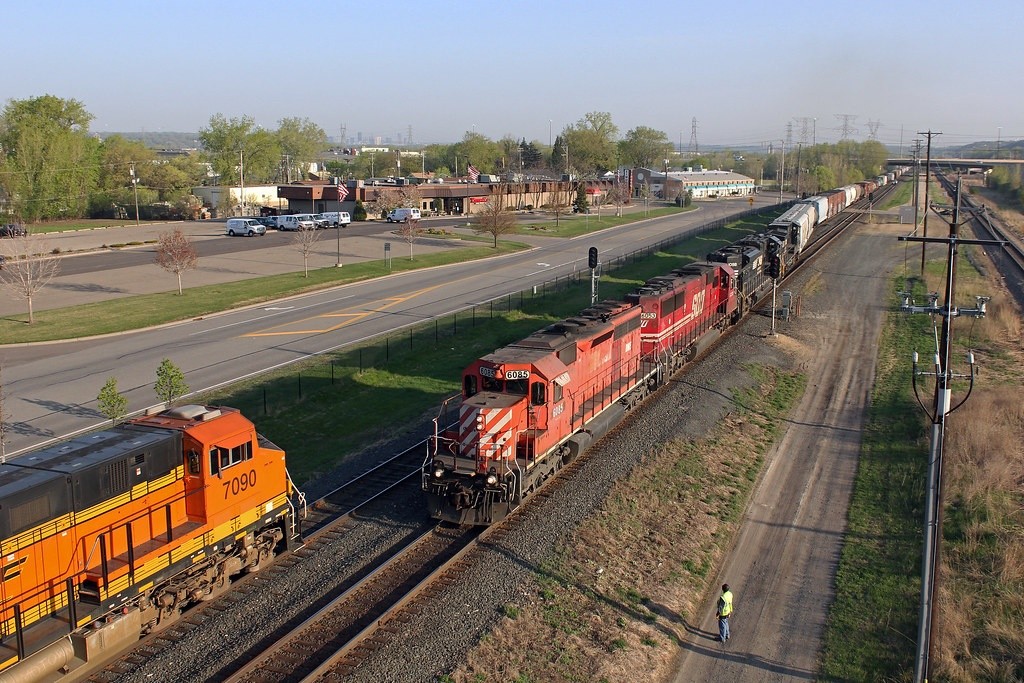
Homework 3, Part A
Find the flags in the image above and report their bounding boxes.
[337,179,349,203]
[468,163,480,179]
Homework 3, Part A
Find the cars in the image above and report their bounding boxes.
[0,225,27,238]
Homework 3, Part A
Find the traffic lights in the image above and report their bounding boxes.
[589,247,597,268]
[772,257,780,277]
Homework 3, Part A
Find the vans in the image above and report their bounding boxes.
[226,219,266,237]
[249,217,277,229]
[386,208,421,223]
[320,212,351,229]
[277,215,315,232]
[268,213,330,230]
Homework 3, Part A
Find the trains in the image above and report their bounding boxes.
[421,164,913,526]
[0,404,307,683]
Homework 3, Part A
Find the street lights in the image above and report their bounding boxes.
[911,353,976,683]
[549,120,552,147]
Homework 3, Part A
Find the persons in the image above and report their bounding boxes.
[715,584,733,642]
